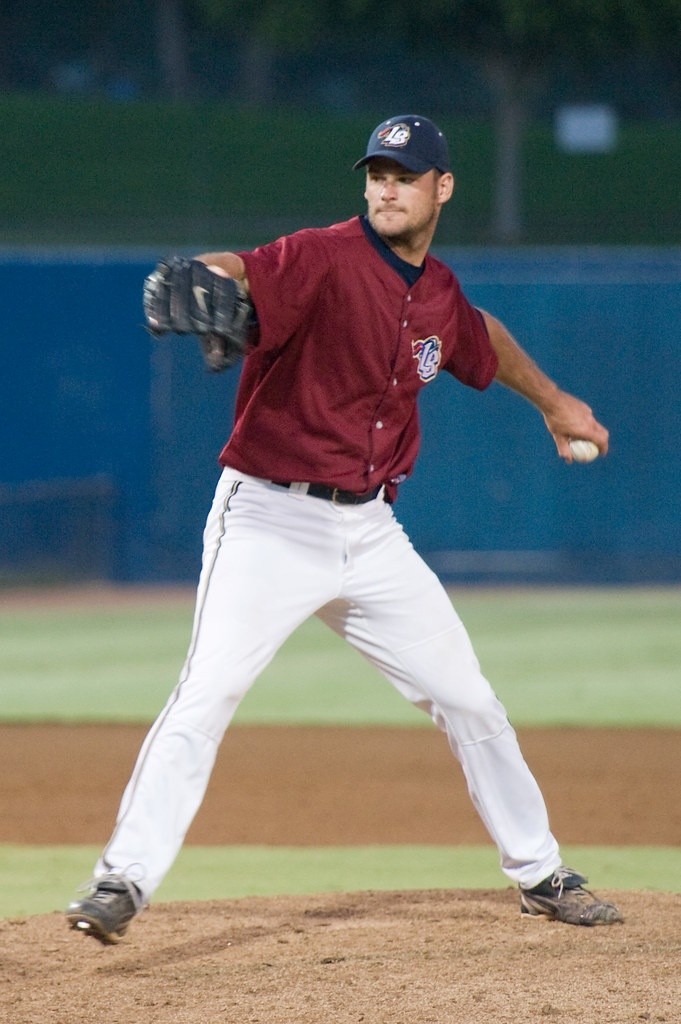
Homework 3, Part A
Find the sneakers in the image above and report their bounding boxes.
[517,865,620,927]
[64,863,149,945]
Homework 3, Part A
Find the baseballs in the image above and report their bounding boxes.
[568,436,600,464]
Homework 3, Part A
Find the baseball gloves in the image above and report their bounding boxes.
[139,252,261,374]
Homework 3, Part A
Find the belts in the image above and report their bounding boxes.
[271,478,384,505]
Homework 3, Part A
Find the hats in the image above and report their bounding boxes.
[352,114,449,174]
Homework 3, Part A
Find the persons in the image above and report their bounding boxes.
[66,114,629,948]
